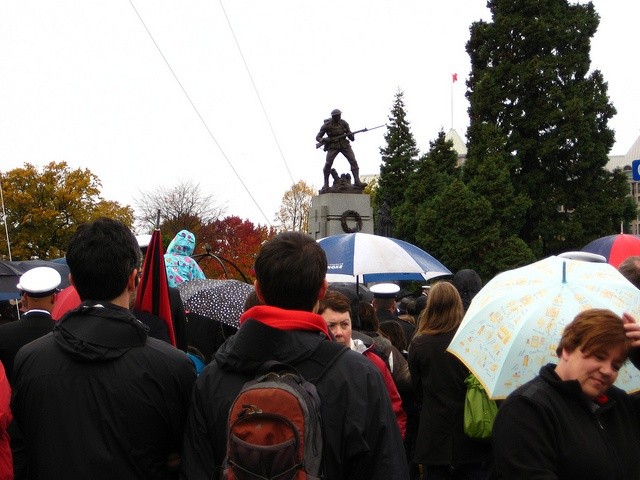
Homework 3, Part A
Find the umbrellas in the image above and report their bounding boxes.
[582,221,640,269]
[177,278,256,330]
[132,209,177,350]
[0,260,53,292]
[316,232,454,297]
[444,255,640,401]
[51,285,82,321]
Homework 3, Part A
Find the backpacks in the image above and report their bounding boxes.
[216,339,351,479]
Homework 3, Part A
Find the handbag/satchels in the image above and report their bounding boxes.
[464,372,498,438]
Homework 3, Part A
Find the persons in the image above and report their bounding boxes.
[368,283,412,344]
[618,256,640,290]
[175,315,226,364]
[491,308,640,480]
[315,109,368,192]
[0,359,15,479]
[318,290,408,444]
[396,298,410,315]
[327,282,412,393]
[176,231,412,479]
[163,229,207,288]
[406,301,417,316]
[10,217,199,478]
[0,267,62,374]
[378,320,408,359]
[416,286,431,308]
[407,282,495,480]
[414,296,428,318]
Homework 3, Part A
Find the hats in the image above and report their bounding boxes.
[20,266,62,297]
[16,284,25,296]
[369,283,400,299]
[421,286,432,290]
[397,297,411,312]
[134,234,152,253]
[558,251,607,262]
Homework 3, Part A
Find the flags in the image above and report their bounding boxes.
[450,73,463,85]
